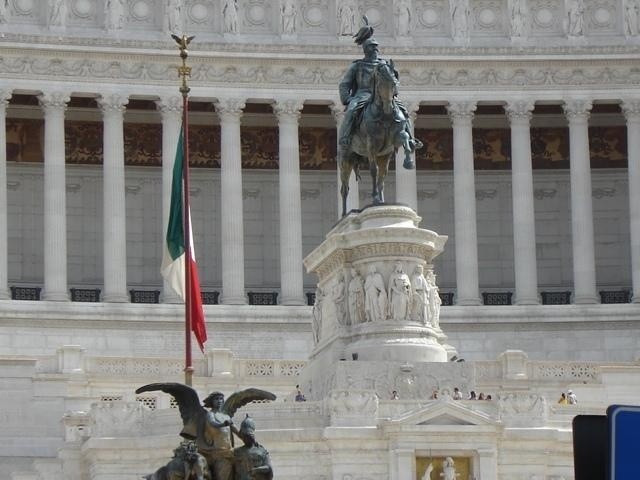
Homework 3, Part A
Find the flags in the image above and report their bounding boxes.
[161,113,208,358]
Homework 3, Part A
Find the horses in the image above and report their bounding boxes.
[337,58,416,219]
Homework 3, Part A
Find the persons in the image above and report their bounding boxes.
[388,260,412,321]
[497,391,550,418]
[328,376,379,417]
[311,287,322,344]
[478,392,485,399]
[339,38,423,151]
[202,391,264,480]
[348,266,365,326]
[453,387,463,399]
[295,390,305,401]
[411,265,429,322]
[567,390,578,404]
[559,392,568,404]
[331,271,347,326]
[487,394,491,400]
[231,412,273,480]
[421,456,461,480]
[365,264,388,323]
[390,391,401,400]
[431,391,439,399]
[469,390,476,400]
[426,271,442,324]
[174,439,212,480]
